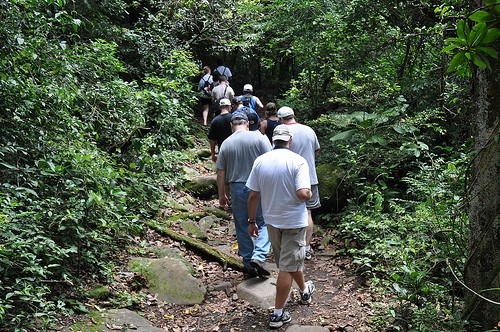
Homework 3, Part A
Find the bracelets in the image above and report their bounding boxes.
[248,219,255,224]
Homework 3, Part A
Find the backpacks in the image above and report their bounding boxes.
[200,76,211,95]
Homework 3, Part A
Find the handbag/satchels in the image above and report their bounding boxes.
[210,99,219,112]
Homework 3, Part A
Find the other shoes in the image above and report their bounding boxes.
[305,245,313,259]
[242,267,257,276]
[249,259,271,277]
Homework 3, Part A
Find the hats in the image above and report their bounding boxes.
[244,84,253,91]
[272,125,292,141]
[247,113,258,130]
[229,113,247,122]
[276,105,294,119]
[220,98,231,106]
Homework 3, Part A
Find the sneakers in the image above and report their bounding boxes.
[299,280,314,304]
[269,311,291,327]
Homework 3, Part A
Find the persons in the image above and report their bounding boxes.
[217,114,272,277]
[260,102,282,146]
[244,125,315,328]
[211,71,221,91]
[208,100,233,162]
[230,98,261,132]
[237,84,263,110]
[212,75,235,100]
[273,106,320,259]
[214,59,232,80]
[198,66,214,126]
